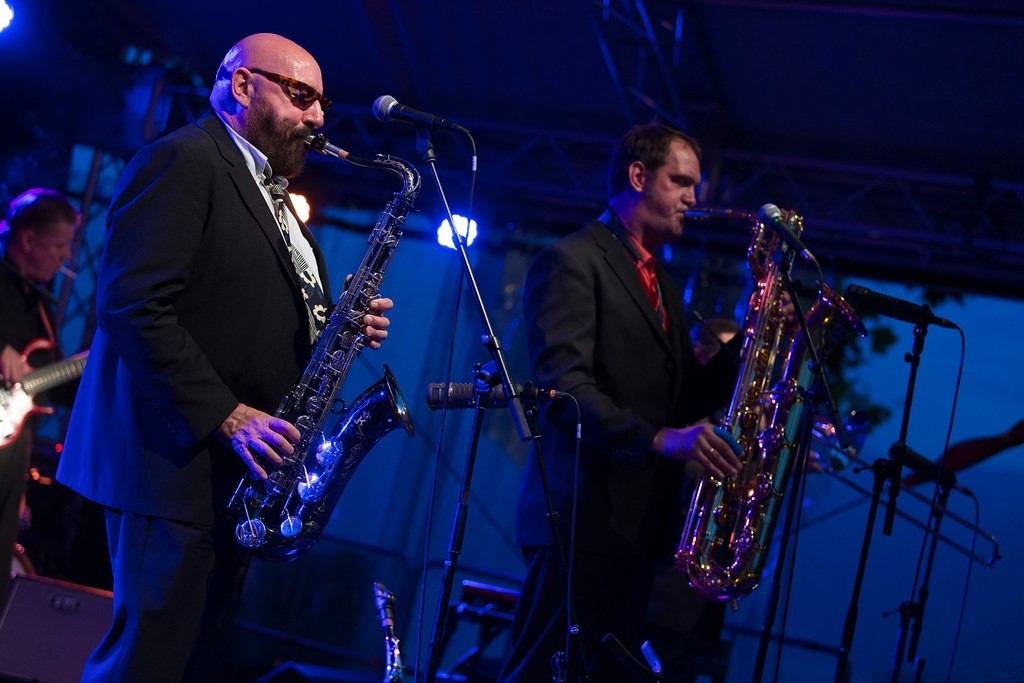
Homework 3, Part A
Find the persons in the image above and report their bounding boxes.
[53,33,394,683]
[692,320,822,472]
[522,124,800,683]
[0,190,72,591]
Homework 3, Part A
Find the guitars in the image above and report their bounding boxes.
[0,341,91,454]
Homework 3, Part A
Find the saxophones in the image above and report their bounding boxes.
[225,131,414,548]
[675,201,870,600]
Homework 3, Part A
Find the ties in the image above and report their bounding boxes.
[260,171,329,331]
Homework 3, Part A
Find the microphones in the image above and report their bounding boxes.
[759,204,815,263]
[847,284,957,329]
[372,94,469,135]
[888,442,971,497]
[426,382,540,410]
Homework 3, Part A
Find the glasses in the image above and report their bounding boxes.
[249,68,333,112]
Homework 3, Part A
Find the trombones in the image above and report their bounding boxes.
[805,417,1001,569]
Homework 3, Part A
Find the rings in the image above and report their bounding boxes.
[707,448,714,456]
